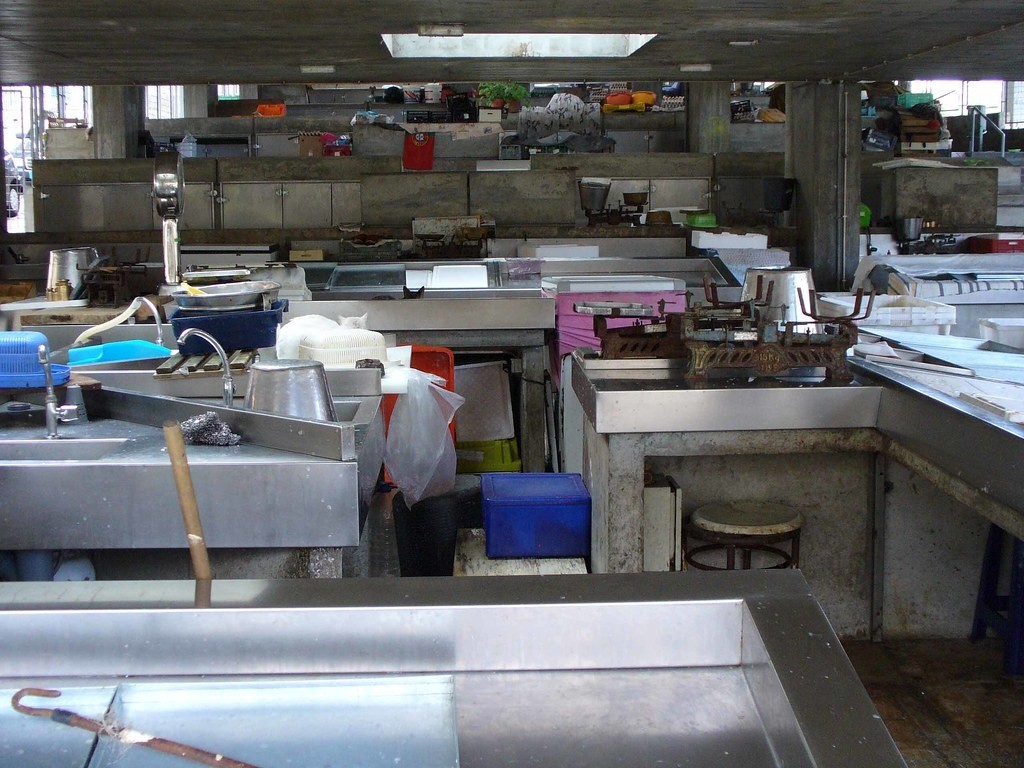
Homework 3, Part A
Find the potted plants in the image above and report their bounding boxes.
[478,81,531,113]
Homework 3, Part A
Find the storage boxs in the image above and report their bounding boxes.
[480,472,591,561]
[978,317,1024,349]
[731,100,754,117]
[381,343,455,483]
[454,438,522,475]
[298,135,351,156]
[969,237,1024,254]
[168,298,289,356]
[897,93,934,110]
[407,93,502,123]
[499,144,615,160]
[540,275,686,390]
[289,249,324,262]
[819,294,956,336]
[862,127,899,152]
[897,139,953,157]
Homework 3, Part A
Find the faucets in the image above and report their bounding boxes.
[35,341,79,438]
[132,296,165,345]
[177,328,237,410]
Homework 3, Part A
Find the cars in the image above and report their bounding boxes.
[10,127,40,182]
[4,148,24,218]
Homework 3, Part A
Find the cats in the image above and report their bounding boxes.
[371,286,424,300]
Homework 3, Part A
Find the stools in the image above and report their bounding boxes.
[682,500,805,571]
[970,522,1024,676]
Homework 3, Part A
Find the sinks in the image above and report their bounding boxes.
[331,395,381,423]
[0,434,130,465]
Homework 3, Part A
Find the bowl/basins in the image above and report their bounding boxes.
[892,216,923,241]
